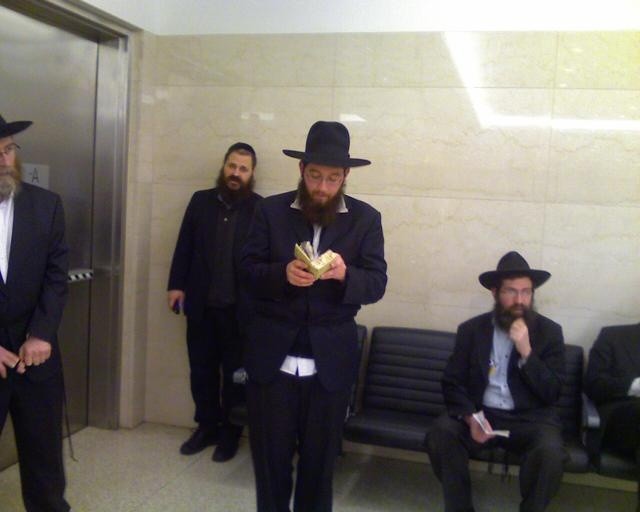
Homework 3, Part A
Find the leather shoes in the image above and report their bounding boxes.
[180,422,216,456]
[212,423,244,462]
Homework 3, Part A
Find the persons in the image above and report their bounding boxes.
[424,252,570,511]
[585,322,640,468]
[167,143,264,463]
[237,121,387,512]
[0,113,70,512]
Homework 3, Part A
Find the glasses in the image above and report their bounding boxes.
[0,143,17,158]
[499,288,532,296]
[305,172,342,185]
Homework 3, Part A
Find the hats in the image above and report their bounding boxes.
[478,252,551,291]
[0,115,33,139]
[282,120,371,168]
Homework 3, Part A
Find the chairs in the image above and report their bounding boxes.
[586,368,639,481]
[469,344,586,479]
[231,321,367,429]
[344,326,459,454]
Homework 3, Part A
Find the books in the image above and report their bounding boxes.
[293,241,335,280]
[473,410,510,438]
